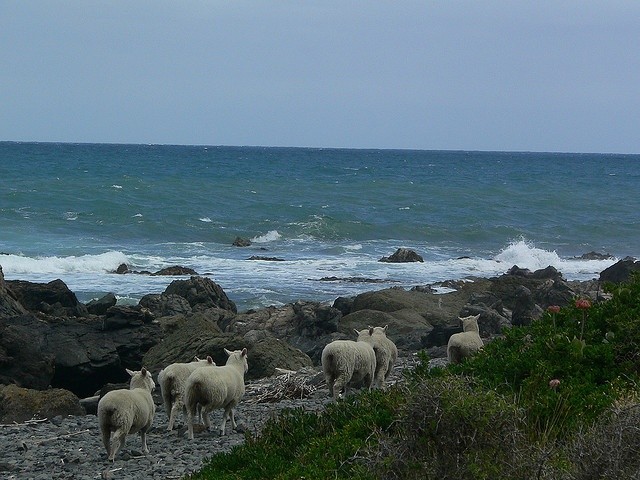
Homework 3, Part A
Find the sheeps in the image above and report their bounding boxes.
[184,347,249,441]
[446,314,485,363]
[366,325,397,390]
[158,356,217,433]
[321,327,377,405]
[97,367,157,464]
